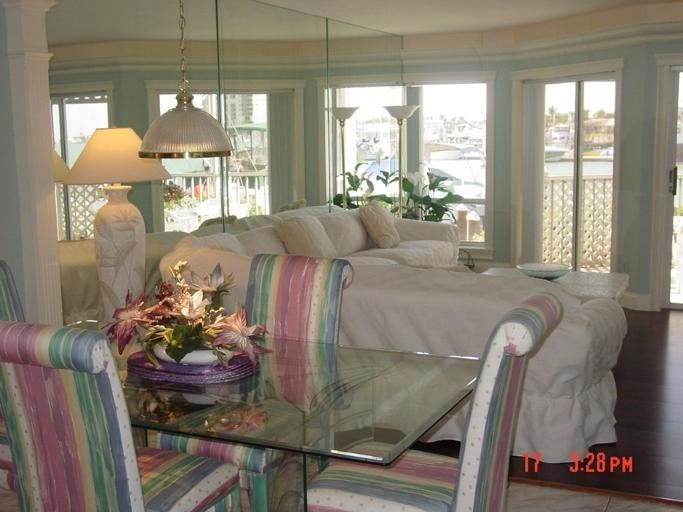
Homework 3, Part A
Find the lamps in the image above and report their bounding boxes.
[64,127,172,324]
[382,104,422,218]
[324,107,359,209]
[139,0,234,159]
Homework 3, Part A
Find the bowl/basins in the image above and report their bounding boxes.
[516,263,569,279]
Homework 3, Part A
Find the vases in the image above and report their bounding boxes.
[154,343,234,365]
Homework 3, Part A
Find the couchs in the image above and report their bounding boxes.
[159,213,627,465]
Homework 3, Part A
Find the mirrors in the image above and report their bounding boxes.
[48,0,405,245]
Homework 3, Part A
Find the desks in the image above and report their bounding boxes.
[70,320,481,512]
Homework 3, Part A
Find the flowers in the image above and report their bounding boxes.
[101,258,272,371]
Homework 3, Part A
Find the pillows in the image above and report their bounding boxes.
[239,203,400,256]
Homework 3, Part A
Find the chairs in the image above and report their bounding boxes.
[0,318,240,512]
[0,259,42,492]
[158,253,352,512]
[306,292,563,511]
